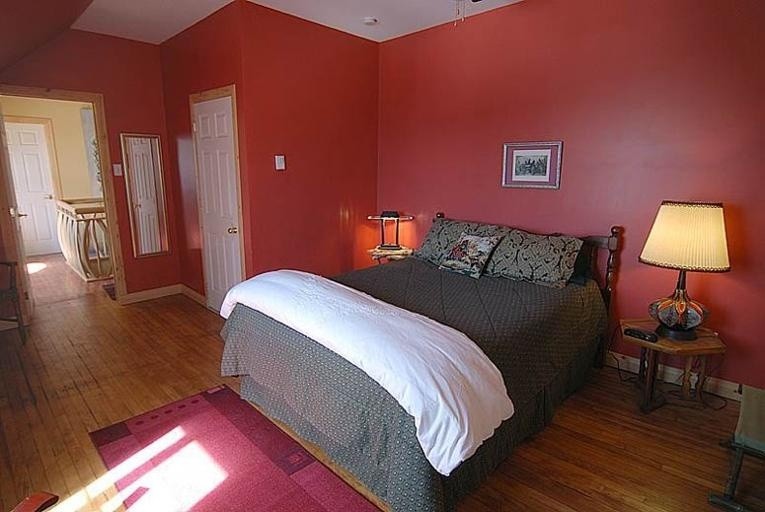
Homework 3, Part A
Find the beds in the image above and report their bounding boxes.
[217,209,621,495]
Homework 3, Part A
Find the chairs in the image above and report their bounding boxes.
[0,261,28,345]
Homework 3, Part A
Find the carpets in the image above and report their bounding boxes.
[86,382,384,512]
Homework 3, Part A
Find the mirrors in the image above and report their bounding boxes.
[120,130,173,261]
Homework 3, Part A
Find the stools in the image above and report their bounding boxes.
[706,382,765,512]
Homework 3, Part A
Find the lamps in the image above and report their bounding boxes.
[636,198,732,343]
[366,211,415,251]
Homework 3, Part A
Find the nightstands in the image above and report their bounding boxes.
[616,317,727,415]
[363,244,413,264]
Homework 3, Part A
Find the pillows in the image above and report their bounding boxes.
[409,214,586,290]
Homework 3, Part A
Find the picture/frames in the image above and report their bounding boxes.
[498,138,565,190]
